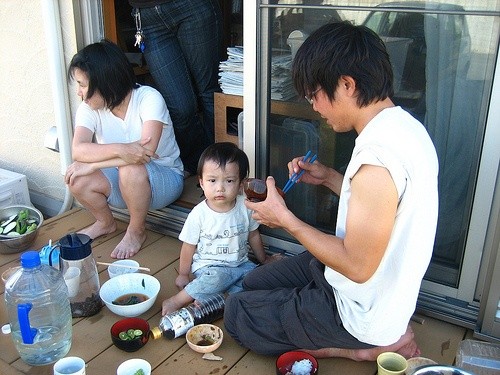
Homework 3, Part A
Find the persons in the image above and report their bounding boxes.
[162,142,285,318]
[64,39,184,260]
[225,20,439,361]
[130,0,223,179]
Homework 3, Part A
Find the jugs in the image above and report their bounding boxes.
[48,231,102,317]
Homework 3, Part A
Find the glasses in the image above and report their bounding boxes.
[305,87,322,104]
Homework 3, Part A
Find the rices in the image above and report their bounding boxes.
[284,359,317,375]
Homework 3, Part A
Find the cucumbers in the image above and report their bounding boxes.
[117,329,143,341]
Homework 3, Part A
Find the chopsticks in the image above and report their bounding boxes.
[282,150,318,194]
[96,261,151,272]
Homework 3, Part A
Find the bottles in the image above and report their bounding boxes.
[4,250,72,365]
[149,292,226,339]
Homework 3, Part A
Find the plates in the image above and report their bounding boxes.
[405,356,436,375]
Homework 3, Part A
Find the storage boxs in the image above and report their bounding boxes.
[286,34,414,95]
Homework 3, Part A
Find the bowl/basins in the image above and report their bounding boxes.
[411,364,475,375]
[99,272,161,316]
[0,204,43,252]
[116,358,152,375]
[241,177,283,204]
[111,317,149,352]
[2,266,22,283]
[186,322,223,354]
[107,259,139,278]
[275,350,319,375]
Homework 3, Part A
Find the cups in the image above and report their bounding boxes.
[377,351,408,374]
[39,238,60,270]
[53,356,85,375]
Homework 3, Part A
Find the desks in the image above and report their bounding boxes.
[213,92,345,225]
